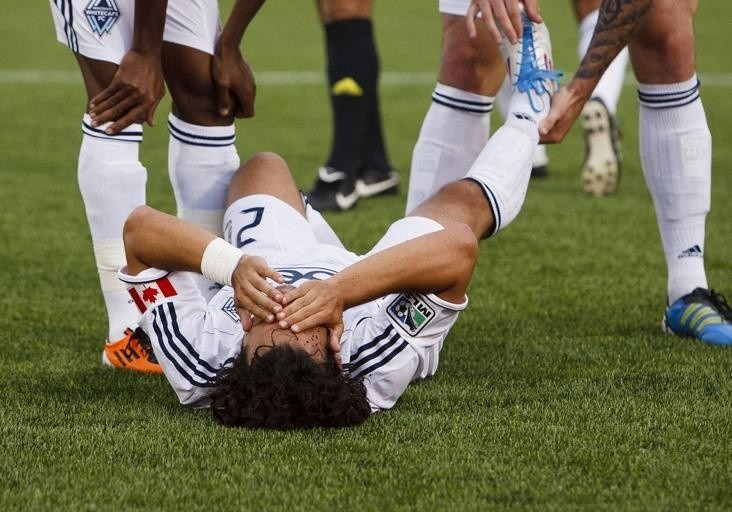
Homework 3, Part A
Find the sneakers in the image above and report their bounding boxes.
[661,288,732,346]
[578,96,620,195]
[303,145,400,211]
[101,327,166,374]
[497,2,558,110]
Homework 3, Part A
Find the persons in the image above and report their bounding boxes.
[399,2,729,346]
[297,2,402,217]
[46,1,266,380]
[491,1,629,201]
[116,2,567,430]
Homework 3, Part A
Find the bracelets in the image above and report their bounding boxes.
[199,235,249,289]
